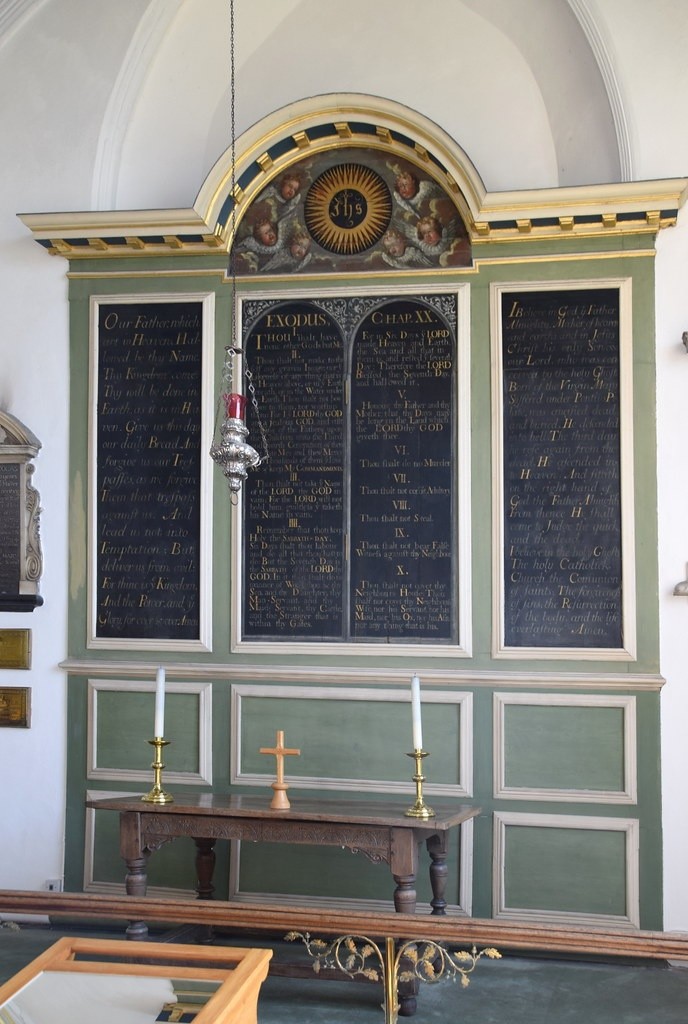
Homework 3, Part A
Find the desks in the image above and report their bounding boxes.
[86,793,483,1015]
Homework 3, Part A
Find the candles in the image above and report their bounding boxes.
[411,678,422,749]
[154,668,165,736]
[223,395,245,418]
